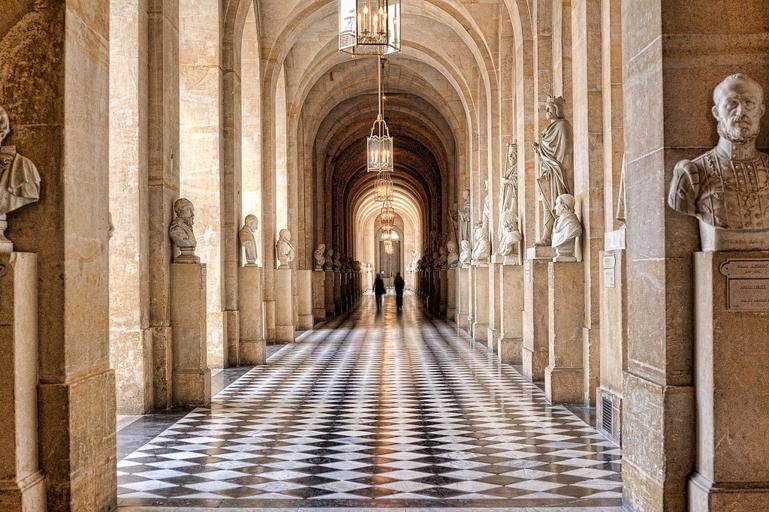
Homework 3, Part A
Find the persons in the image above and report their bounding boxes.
[239,214,257,264]
[276,228,297,269]
[168,197,198,256]
[393,272,405,311]
[420,92,583,268]
[372,273,385,312]
[314,242,369,271]
[666,70,769,253]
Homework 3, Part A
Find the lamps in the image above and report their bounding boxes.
[375,172,394,247]
[337,0,402,56]
[367,55,394,172]
